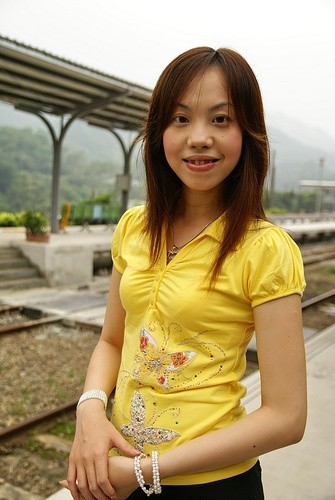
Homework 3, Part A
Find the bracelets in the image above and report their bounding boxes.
[134,451,162,496]
[77,390,107,410]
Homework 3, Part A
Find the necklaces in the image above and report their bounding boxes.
[167,205,229,264]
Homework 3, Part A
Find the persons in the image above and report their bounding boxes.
[60,47,307,500]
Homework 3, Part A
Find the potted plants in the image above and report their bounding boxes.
[23,212,49,243]
